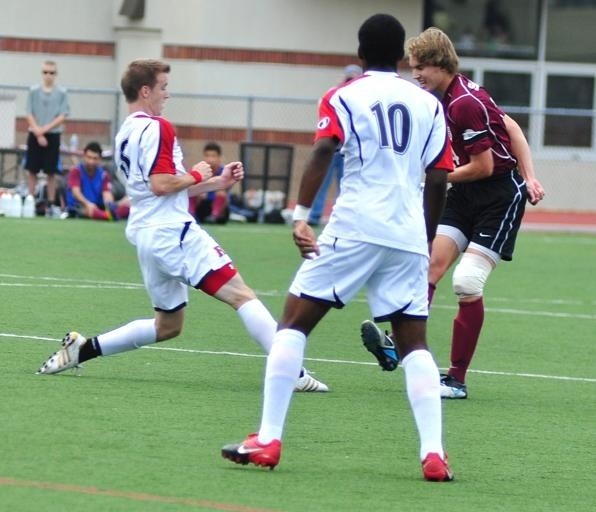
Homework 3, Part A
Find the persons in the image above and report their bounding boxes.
[64,138,129,221]
[217,14,457,485]
[358,27,551,402]
[22,55,71,212]
[32,57,332,396]
[184,141,232,222]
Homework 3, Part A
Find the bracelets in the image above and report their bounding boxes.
[188,167,205,183]
[292,203,313,222]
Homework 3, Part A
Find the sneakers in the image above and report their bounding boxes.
[222,433,281,469]
[36,332,86,374]
[440,373,467,398]
[361,320,399,370]
[422,451,453,481]
[297,368,329,392]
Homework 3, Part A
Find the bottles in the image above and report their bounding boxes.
[0,193,35,218]
[70,133,77,151]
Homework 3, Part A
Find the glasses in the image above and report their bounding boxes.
[44,69,55,75]
